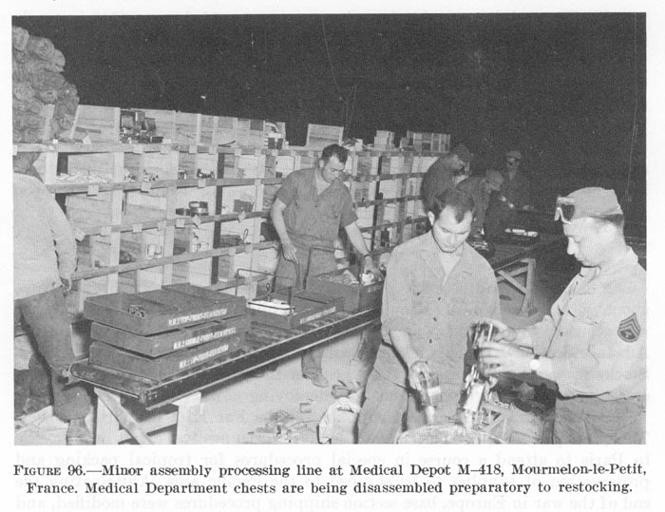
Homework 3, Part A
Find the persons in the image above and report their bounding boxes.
[265,144,379,387]
[484,150,530,244]
[13,149,92,445]
[355,189,502,444]
[468,186,644,443]
[419,143,471,231]
[459,168,504,239]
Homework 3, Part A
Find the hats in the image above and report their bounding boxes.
[486,169,504,187]
[555,187,624,218]
[455,145,472,162]
[505,150,522,160]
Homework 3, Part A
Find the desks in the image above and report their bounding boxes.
[71,235,566,444]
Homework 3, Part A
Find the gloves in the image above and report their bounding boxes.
[409,360,442,408]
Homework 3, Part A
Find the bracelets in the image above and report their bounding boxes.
[364,253,372,258]
[410,359,425,368]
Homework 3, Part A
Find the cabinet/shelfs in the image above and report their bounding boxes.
[13,142,450,359]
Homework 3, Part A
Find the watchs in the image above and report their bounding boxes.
[531,353,540,380]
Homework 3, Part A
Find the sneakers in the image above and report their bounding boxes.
[303,371,329,387]
[66,426,95,445]
